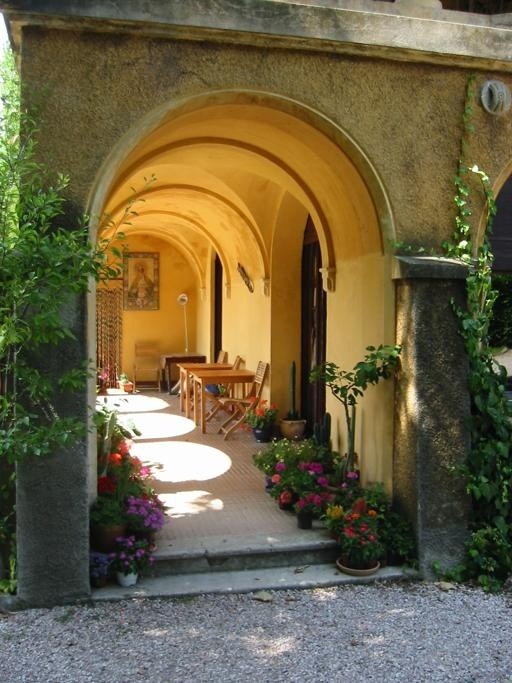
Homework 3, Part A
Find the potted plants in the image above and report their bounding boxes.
[88,495,128,552]
[279,361,307,441]
[118,375,134,392]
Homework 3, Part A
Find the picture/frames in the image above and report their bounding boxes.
[122,252,160,311]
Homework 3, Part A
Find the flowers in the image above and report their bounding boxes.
[331,508,385,566]
[90,552,111,579]
[254,435,336,512]
[109,536,155,577]
[239,396,278,432]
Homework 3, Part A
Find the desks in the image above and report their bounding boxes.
[162,355,207,395]
[188,370,256,434]
[178,362,233,418]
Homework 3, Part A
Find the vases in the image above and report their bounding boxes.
[265,476,312,529]
[337,534,379,570]
[252,427,273,443]
[116,570,138,588]
[95,574,107,587]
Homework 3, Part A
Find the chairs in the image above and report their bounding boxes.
[190,350,228,411]
[204,355,242,422]
[217,361,270,441]
